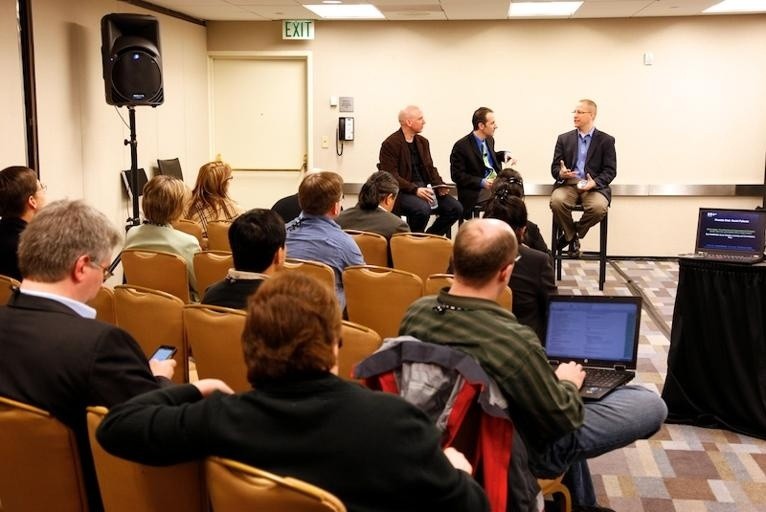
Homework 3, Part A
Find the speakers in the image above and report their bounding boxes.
[100,12,165,108]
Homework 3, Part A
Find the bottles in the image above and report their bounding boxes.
[427,184,439,210]
[576,178,587,189]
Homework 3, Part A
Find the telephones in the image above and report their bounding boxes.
[338,117,354,141]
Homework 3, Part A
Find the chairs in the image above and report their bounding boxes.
[283,258,513,338]
[120,250,234,303]
[1,275,22,305]
[337,320,382,381]
[536,476,572,512]
[174,220,232,251]
[85,285,252,391]
[375,162,453,243]
[1,398,346,512]
[343,230,451,276]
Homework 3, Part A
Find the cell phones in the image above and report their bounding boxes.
[148,344,177,361]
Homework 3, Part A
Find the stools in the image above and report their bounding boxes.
[545,202,609,293]
[456,203,487,229]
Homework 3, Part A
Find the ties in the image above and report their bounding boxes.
[481,142,498,181]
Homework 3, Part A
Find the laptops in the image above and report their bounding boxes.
[540,294,642,401]
[675,208,766,264]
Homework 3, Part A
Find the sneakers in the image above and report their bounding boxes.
[557,232,567,251]
[568,235,581,257]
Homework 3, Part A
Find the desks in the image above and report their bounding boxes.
[659,248,766,445]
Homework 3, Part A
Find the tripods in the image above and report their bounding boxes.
[103,105,141,285]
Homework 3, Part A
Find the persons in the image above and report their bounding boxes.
[450,107,517,220]
[379,104,464,236]
[550,98,616,259]
